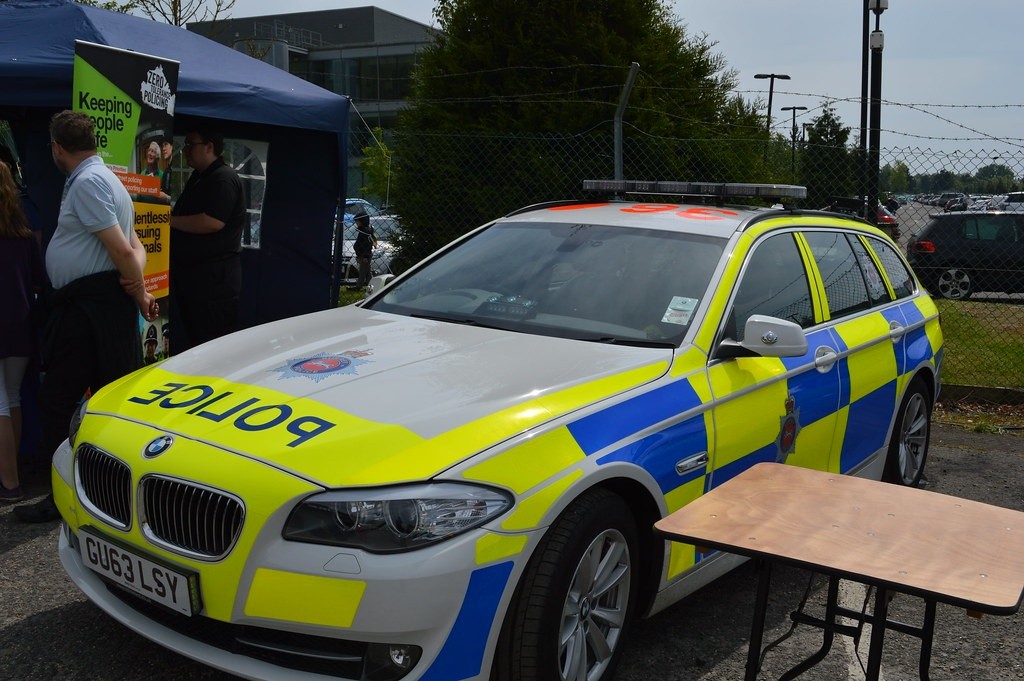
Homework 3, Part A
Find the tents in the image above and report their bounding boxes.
[0,0,351,457]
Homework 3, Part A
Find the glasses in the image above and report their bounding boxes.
[184,139,208,146]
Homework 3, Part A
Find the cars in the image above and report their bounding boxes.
[883,191,1024,214]
[333,214,406,288]
[46,178,948,681]
[901,210,1024,302]
[817,197,902,240]
[331,198,384,227]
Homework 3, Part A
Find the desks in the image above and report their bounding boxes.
[653,462,1024,681]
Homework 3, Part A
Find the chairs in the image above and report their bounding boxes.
[996,226,1015,241]
[551,242,626,322]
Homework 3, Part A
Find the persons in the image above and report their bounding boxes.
[137,136,171,202]
[42,110,160,394]
[170,121,246,346]
[0,160,40,500]
[143,323,169,366]
[354,213,373,287]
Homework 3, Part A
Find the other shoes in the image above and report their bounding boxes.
[0,482,23,502]
[13,495,62,522]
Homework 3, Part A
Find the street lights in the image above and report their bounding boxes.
[754,74,792,170]
[781,106,809,173]
